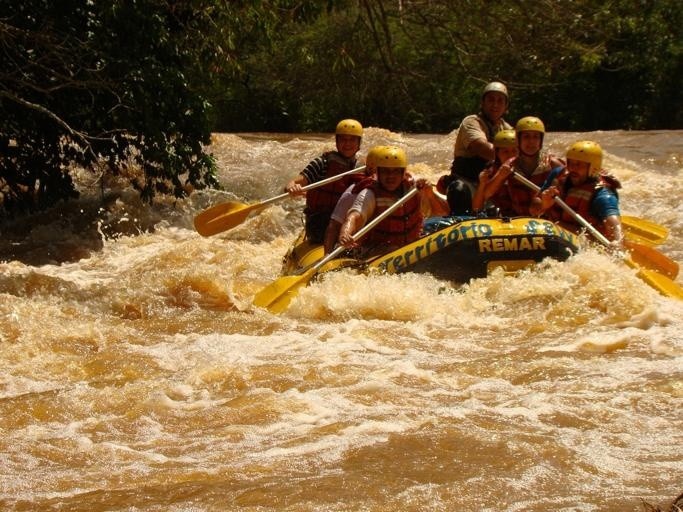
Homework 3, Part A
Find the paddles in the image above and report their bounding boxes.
[194,164,367,239]
[255,185,419,315]
[506,167,683,301]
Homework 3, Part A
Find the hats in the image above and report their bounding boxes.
[481,82,509,105]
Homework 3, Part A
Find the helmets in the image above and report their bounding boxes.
[515,117,545,148]
[335,119,361,148]
[366,146,406,177]
[495,130,518,148]
[566,141,602,178]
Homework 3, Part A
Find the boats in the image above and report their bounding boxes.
[280,213,585,293]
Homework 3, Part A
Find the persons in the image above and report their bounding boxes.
[446,81,515,217]
[471,130,520,217]
[481,116,567,223]
[338,146,434,260]
[284,118,367,245]
[528,140,624,253]
[324,146,380,261]
